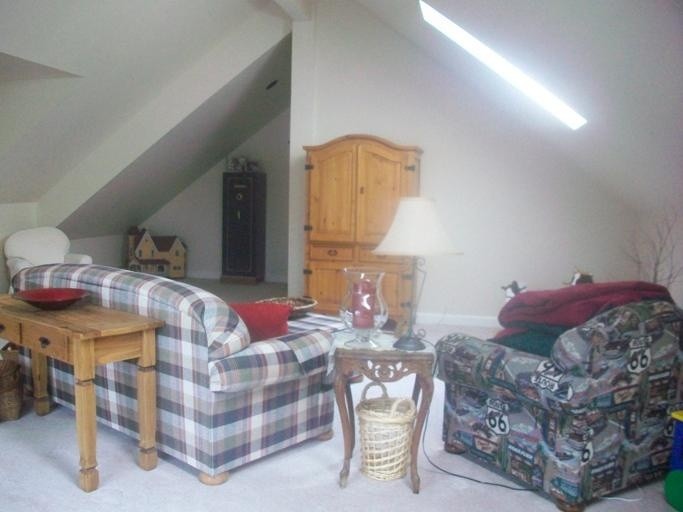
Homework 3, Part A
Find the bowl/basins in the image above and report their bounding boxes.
[12,285,90,311]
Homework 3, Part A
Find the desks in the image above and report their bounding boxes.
[0,295,165,493]
[287,312,348,335]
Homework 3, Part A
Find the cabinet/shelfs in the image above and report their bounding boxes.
[221,172,266,284]
[303,134,422,331]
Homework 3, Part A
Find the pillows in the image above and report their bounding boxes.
[230,303,293,342]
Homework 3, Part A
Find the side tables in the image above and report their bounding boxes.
[330,334,435,494]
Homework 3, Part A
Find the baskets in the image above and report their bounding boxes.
[356,397,417,482]
[0,352,22,421]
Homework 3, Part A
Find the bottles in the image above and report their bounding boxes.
[341,264,391,351]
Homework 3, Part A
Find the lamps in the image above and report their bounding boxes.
[371,197,464,350]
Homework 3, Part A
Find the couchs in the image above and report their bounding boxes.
[12,263,338,485]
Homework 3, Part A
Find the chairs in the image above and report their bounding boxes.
[4,227,92,295]
[435,299,683,512]
